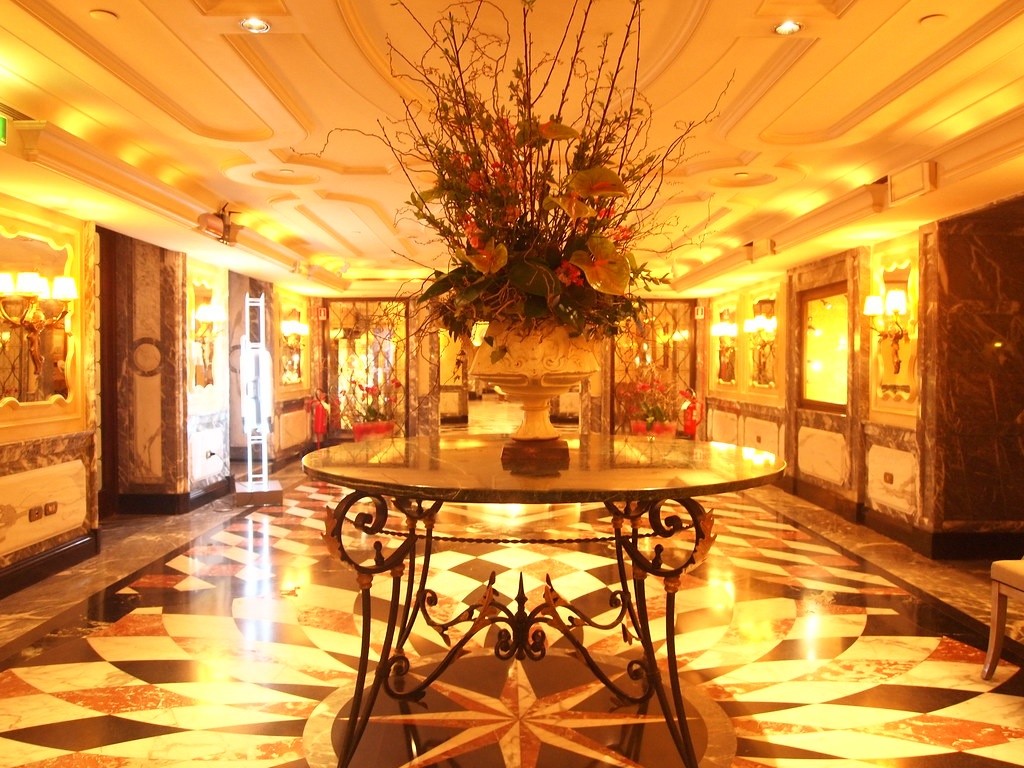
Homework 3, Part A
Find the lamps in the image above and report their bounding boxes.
[1,271,81,373]
[865,289,907,375]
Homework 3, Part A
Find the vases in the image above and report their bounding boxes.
[630,420,677,439]
[353,423,395,441]
[471,311,600,440]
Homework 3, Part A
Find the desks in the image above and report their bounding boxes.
[301,434,787,768]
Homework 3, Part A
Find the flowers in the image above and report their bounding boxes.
[341,367,403,424]
[620,378,697,431]
[292,0,736,365]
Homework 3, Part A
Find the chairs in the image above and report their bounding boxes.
[981,560,1024,679]
